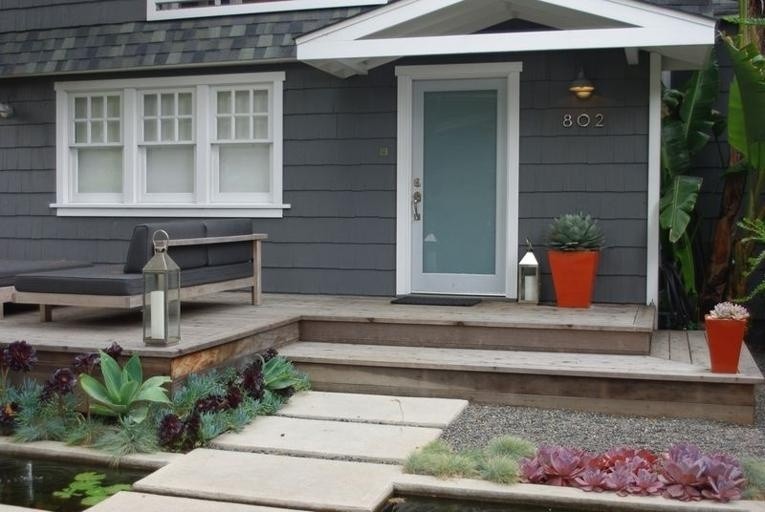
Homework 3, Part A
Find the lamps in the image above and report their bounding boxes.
[569,50,595,98]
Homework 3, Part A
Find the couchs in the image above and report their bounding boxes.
[1,219,268,323]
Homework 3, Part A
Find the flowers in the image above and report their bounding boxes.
[710,300,751,321]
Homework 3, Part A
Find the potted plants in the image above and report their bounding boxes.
[545,210,606,308]
[704,315,750,374]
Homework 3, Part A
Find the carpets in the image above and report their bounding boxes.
[390,296,482,307]
